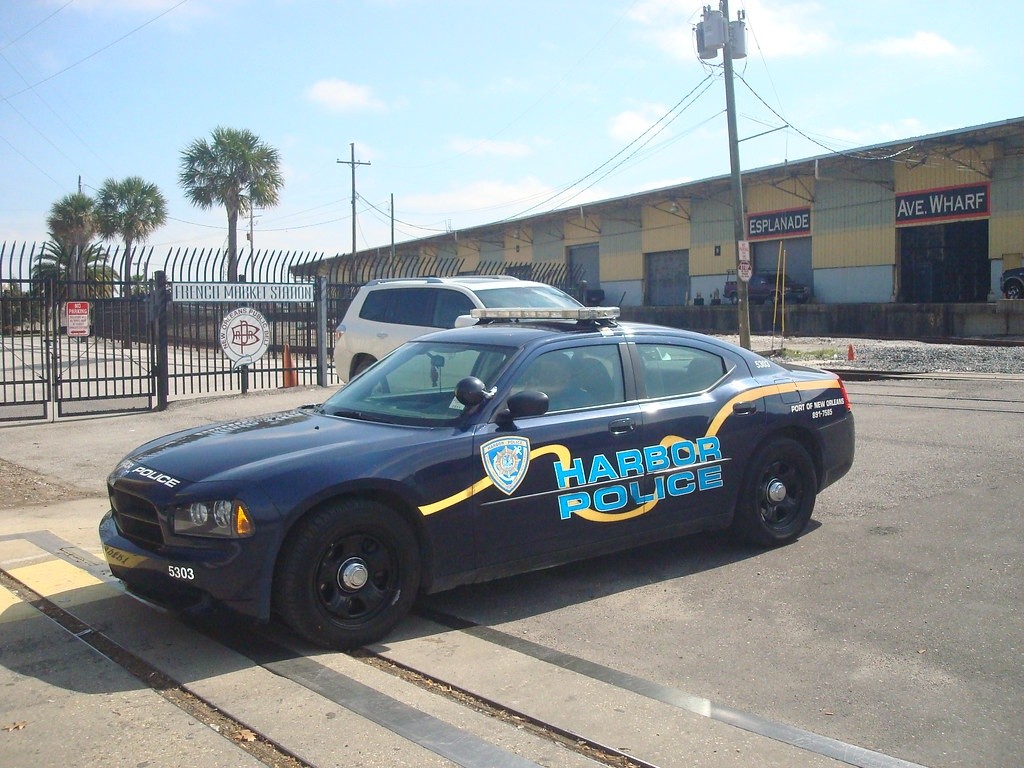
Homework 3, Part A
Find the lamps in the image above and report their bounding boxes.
[514,229,520,240]
[670,197,679,212]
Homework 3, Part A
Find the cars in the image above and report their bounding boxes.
[96,312,855,653]
[1000,266,1024,299]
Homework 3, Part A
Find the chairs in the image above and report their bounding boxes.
[678,356,720,392]
[579,358,611,402]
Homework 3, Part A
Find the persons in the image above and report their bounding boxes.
[535,353,593,410]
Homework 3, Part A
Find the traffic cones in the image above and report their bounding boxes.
[280,343,298,389]
[847,345,855,361]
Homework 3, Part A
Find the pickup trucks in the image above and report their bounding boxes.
[552,280,605,307]
[722,268,809,306]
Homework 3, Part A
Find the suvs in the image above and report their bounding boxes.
[332,275,588,396]
